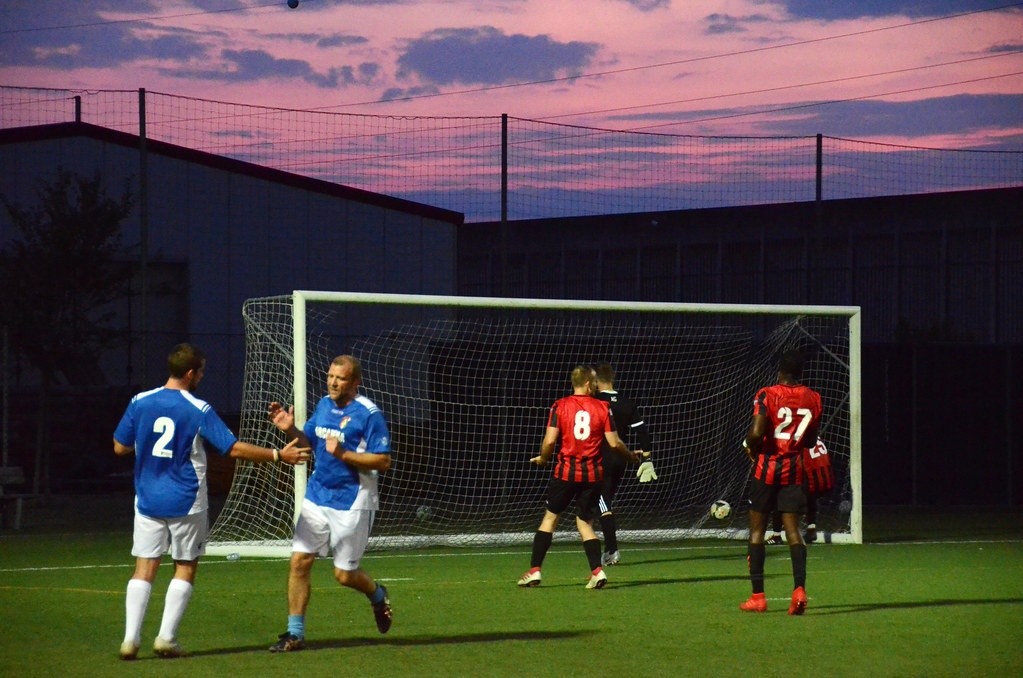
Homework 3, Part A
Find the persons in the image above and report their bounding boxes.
[517,366,645,589]
[266,354,394,652]
[763,434,834,545]
[112,343,312,658]
[597,363,659,566]
[739,350,824,616]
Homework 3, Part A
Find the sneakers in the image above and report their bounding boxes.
[119,641,139,659]
[153,636,194,657]
[517,567,542,587]
[601,549,620,566]
[365,582,393,634]
[803,528,817,543]
[788,586,807,616]
[739,593,767,612]
[269,632,306,652]
[764,534,783,545]
[585,567,607,589]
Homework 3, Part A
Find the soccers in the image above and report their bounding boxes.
[710,500,731,520]
[417,505,432,520]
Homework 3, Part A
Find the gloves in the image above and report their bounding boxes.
[636,457,657,483]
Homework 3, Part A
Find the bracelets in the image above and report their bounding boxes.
[743,437,748,449]
[273,448,282,463]
